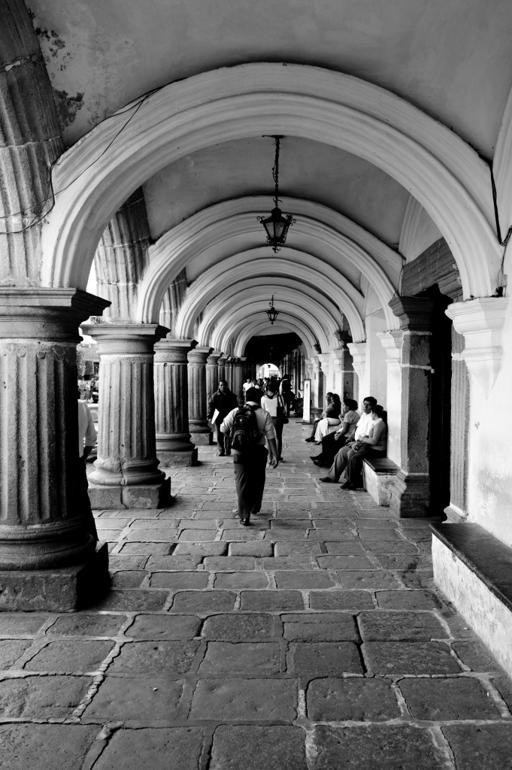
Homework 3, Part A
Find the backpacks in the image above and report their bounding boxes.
[229,403,261,451]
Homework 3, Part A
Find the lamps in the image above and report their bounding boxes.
[256,134,296,253]
[264,294,279,326]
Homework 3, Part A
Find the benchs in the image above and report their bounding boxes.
[428,517,511,677]
[361,455,399,507]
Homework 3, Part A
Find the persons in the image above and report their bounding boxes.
[315,394,341,445]
[311,399,361,467]
[319,396,377,484]
[78,399,98,489]
[219,388,279,524]
[207,379,239,456]
[242,374,295,416]
[340,405,387,490]
[304,392,333,442]
[261,385,284,465]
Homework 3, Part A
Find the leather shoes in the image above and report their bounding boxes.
[240,517,250,526]
[319,477,338,483]
[340,481,356,490]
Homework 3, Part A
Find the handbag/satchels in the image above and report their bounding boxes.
[276,395,289,424]
